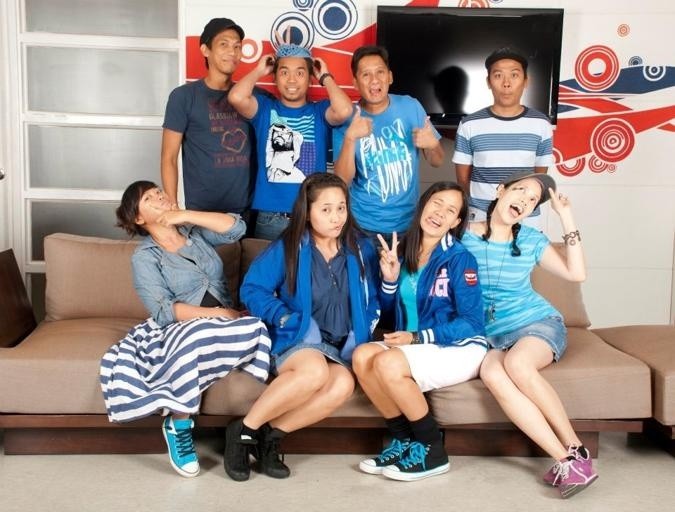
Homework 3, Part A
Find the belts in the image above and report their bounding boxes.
[275,212,291,218]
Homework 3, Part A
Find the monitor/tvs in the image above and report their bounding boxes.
[375,5,564,135]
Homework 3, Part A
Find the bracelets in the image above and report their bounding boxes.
[319,72,334,87]
[562,229,581,244]
[412,331,422,344]
[280,314,291,328]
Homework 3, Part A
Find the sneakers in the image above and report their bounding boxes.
[544,444,600,498]
[224,418,290,480]
[359,431,450,481]
[161,415,201,477]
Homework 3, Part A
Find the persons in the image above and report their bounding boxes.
[461,173,600,498]
[114,179,247,476]
[352,178,488,482]
[329,42,446,245]
[222,171,381,481]
[227,42,354,241]
[451,45,554,233]
[159,17,277,239]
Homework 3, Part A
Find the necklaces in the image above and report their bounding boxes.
[481,230,510,321]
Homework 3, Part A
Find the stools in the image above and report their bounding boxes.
[598,325,675,440]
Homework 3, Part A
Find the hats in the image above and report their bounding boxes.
[277,44,311,58]
[200,18,244,43]
[496,171,557,205]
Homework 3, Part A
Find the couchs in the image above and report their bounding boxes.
[0,233,652,458]
